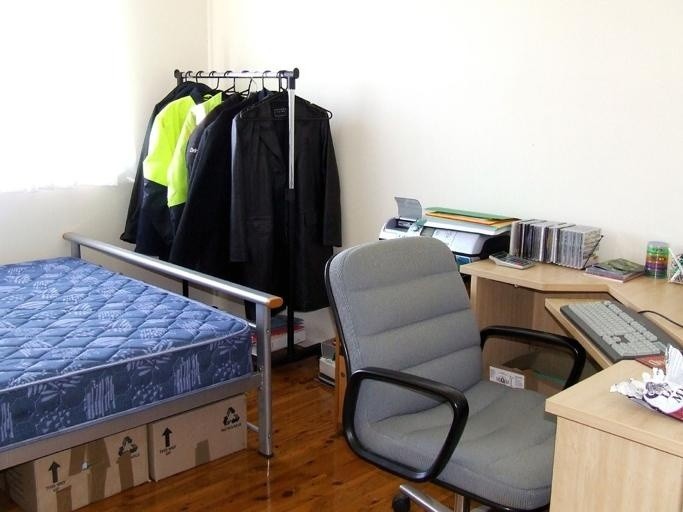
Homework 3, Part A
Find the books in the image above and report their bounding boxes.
[583,255,647,283]
[417,206,521,237]
[508,219,601,271]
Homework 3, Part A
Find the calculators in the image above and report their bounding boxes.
[489,251,535,270]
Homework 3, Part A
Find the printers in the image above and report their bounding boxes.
[378,196,509,267]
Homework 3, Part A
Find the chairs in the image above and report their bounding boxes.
[323,236,586,511]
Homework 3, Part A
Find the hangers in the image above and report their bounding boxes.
[180,71,334,122]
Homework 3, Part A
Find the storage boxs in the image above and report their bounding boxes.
[148,391,248,483]
[4,426,151,512]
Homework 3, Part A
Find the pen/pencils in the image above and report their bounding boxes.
[668,248,683,281]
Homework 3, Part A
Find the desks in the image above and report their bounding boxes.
[458,254,682,512]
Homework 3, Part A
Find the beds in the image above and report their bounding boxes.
[0,232,283,489]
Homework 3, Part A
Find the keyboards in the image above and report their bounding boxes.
[561,299,683,364]
